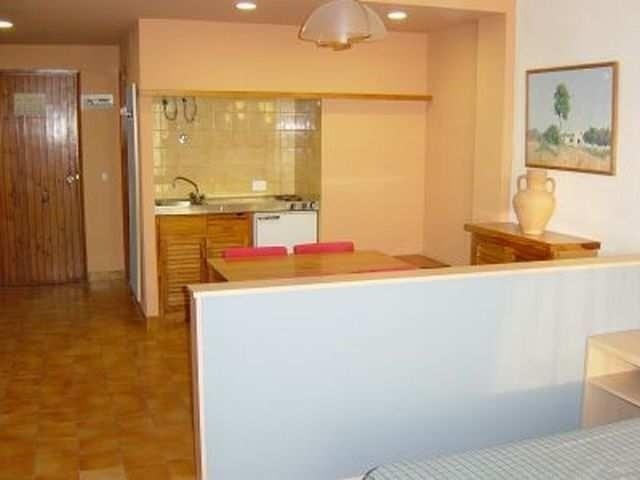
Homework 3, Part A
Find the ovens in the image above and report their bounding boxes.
[253,202,319,254]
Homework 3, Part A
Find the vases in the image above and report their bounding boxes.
[511,168,556,236]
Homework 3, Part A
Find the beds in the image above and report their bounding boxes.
[359,415,640,480]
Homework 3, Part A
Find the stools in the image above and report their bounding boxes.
[395,255,448,267]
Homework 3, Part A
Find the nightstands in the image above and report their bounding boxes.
[580,327,640,429]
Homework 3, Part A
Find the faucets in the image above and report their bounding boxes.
[171,176,207,205]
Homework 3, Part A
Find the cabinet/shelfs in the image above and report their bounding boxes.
[155,215,251,318]
[464,222,600,264]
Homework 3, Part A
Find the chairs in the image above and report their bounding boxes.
[295,241,353,253]
[223,246,287,257]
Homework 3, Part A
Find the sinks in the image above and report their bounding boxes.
[155,199,207,206]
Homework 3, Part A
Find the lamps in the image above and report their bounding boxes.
[298,0,386,51]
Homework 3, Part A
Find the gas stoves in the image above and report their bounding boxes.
[254,195,312,201]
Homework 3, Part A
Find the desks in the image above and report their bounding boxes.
[207,249,420,282]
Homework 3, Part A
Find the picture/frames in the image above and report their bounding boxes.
[524,61,617,176]
